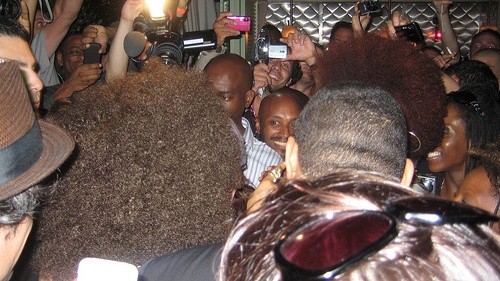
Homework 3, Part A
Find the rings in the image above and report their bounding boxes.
[267,165,283,183]
[223,23,226,27]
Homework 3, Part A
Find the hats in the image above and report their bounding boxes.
[0,56,76,202]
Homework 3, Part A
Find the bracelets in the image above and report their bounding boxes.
[176,7,185,13]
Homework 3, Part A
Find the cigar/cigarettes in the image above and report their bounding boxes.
[446,45,455,59]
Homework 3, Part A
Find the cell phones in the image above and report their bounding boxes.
[226,16,250,31]
[394,22,425,44]
[83,42,101,65]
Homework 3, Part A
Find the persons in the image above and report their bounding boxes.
[0,0,500,281]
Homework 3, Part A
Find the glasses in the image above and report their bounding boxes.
[274,195,500,281]
[446,91,485,117]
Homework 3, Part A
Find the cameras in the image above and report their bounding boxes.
[411,173,437,194]
[358,0,382,15]
[254,28,287,65]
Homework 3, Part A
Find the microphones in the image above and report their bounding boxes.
[123,32,147,58]
